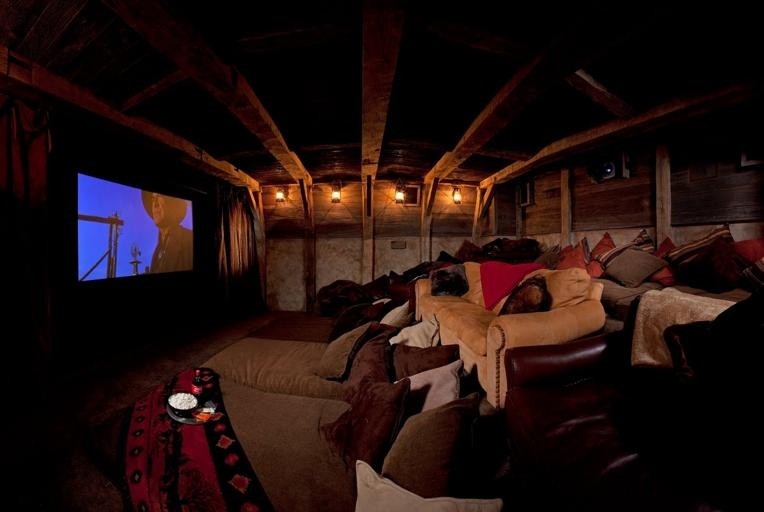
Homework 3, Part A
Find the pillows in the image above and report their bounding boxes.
[312,227,763,511]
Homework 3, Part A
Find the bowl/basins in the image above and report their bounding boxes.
[167,392,200,418]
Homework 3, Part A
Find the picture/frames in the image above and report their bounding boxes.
[402,185,421,208]
[518,179,534,209]
[740,146,763,168]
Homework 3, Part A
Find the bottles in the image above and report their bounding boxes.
[191,368,203,405]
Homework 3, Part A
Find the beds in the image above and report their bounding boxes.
[95,336,467,512]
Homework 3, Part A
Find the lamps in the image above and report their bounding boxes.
[392,180,405,204]
[272,180,285,201]
[451,180,464,204]
[330,179,341,207]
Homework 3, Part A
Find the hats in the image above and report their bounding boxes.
[140,190,189,228]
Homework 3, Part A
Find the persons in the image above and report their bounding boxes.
[140,191,193,271]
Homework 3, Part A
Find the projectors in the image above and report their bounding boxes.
[593,158,629,181]
